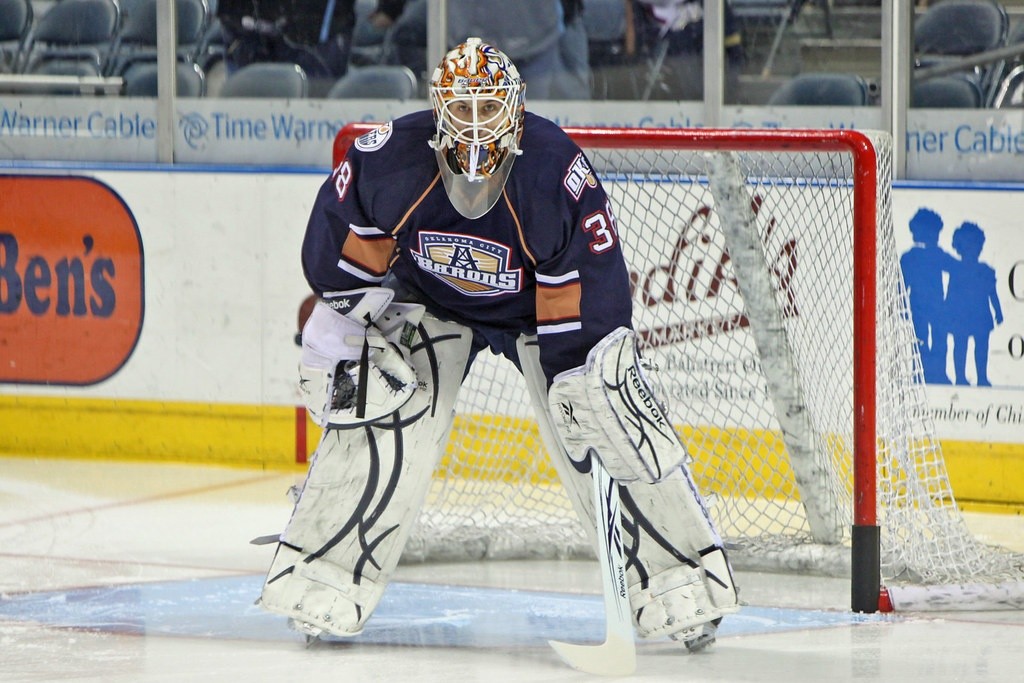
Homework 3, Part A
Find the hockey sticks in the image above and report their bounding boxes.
[545,447,639,679]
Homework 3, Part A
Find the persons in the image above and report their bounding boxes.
[255,39,742,651]
[215,0,747,101]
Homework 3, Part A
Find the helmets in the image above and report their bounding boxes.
[428,36,527,183]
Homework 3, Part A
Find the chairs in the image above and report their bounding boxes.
[0,0,1024,110]
